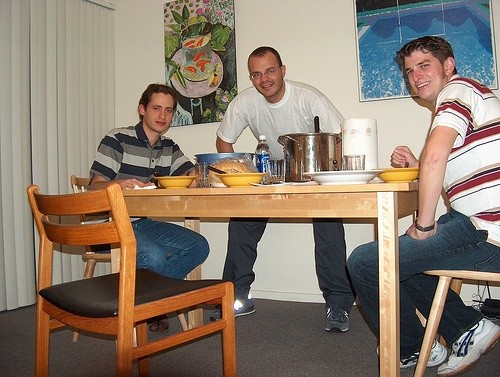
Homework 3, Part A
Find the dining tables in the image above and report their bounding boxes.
[111,180,419,377]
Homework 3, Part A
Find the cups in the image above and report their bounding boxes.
[269,159,285,184]
[342,154,366,170]
[194,162,212,188]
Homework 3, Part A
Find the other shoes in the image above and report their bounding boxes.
[146,313,169,334]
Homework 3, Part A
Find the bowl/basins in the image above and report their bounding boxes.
[373,168,420,183]
[216,172,264,187]
[154,175,196,189]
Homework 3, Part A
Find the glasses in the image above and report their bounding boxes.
[249,66,280,82]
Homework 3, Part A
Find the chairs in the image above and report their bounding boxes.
[70,174,187,343]
[27,183,236,377]
[414,270,500,377]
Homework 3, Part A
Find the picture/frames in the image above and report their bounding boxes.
[354,0,500,102]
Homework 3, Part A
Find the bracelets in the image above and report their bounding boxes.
[415,219,435,232]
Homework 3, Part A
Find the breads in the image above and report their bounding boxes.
[208,162,251,183]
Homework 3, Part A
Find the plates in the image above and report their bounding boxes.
[303,170,384,184]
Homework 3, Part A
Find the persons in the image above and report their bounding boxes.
[85,84,210,333]
[346,35,500,377]
[210,46,356,332]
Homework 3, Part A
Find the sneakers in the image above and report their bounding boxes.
[209,298,256,321]
[437,317,500,377]
[325,305,350,333]
[377,339,447,368]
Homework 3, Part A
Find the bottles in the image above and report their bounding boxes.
[255,135,272,173]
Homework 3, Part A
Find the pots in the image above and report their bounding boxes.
[194,152,256,188]
[277,132,343,183]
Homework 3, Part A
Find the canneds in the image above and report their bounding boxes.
[255,150,270,185]
